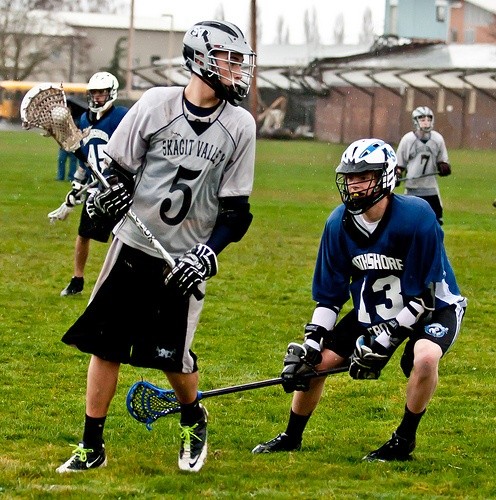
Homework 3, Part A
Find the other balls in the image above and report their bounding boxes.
[51,106,70,126]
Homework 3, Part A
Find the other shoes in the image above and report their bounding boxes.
[55,177,64,181]
[67,178,74,182]
[60,276,84,296]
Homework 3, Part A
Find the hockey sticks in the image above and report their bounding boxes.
[126,365,351,425]
[394,172,440,182]
[47,178,98,222]
[19,82,206,303]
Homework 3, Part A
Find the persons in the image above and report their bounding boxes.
[253,138,468,460]
[55,148,76,183]
[394,107,451,224]
[53,21,257,472]
[60,71,129,295]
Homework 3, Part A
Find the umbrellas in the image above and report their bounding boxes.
[66,95,89,120]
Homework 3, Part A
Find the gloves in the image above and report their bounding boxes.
[280,338,323,393]
[396,172,403,188]
[86,175,133,231]
[436,161,451,178]
[161,242,219,303]
[349,335,390,380]
[64,189,85,208]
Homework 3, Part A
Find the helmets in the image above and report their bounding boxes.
[86,71,120,113]
[412,106,435,134]
[180,19,257,108]
[335,139,398,215]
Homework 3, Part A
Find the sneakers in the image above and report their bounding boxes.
[362,431,417,463]
[55,441,108,473]
[178,403,209,472]
[252,432,303,454]
